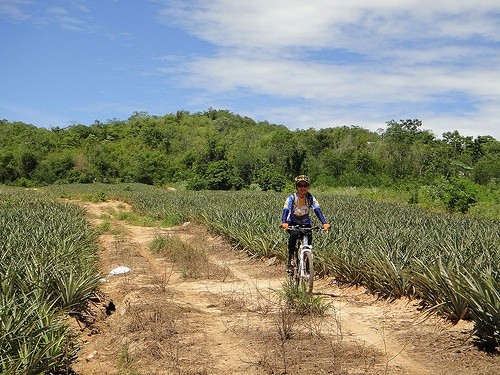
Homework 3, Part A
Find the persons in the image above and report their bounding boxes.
[281,175,330,274]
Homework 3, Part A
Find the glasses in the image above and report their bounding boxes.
[296,183,309,188]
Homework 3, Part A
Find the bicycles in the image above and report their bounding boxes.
[279,224,332,294]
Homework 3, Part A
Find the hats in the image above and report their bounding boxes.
[297,175,310,185]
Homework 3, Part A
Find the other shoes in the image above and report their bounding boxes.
[286,267,295,272]
[305,269,316,275]
